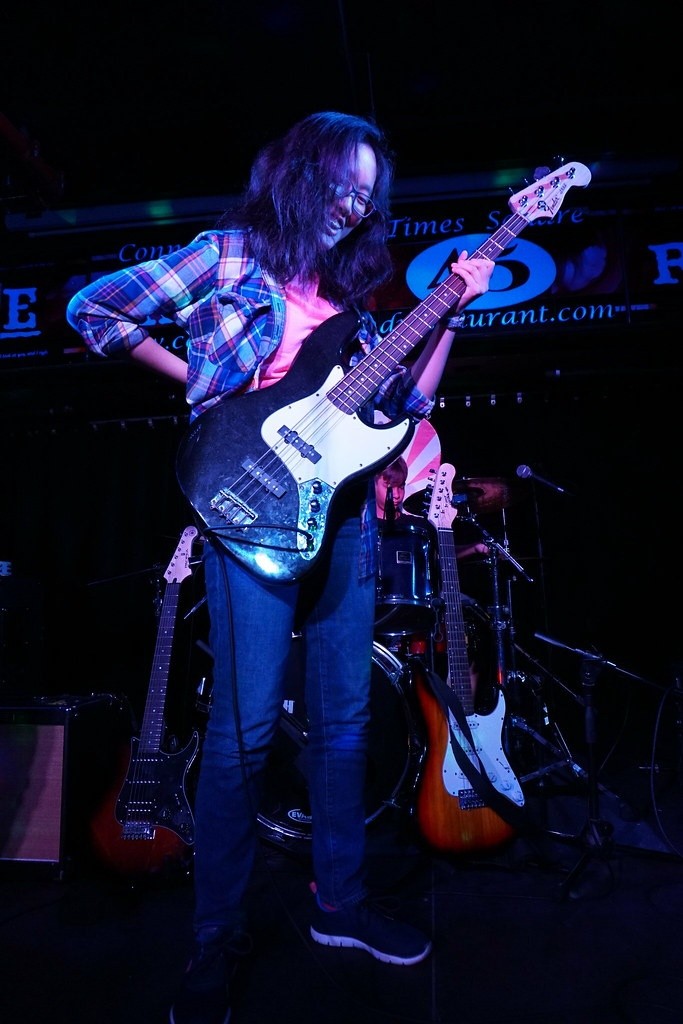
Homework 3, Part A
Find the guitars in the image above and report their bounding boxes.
[174,161,592,585]
[88,526,201,882]
[410,461,529,859]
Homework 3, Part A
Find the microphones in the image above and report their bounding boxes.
[385,487,395,520]
[517,464,569,496]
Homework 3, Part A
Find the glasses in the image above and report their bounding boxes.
[324,171,377,218]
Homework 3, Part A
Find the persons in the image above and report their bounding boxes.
[66,113,495,1024]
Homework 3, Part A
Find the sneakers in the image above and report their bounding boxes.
[309,893,432,967]
[170,930,237,1023]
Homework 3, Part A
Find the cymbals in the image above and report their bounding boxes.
[402,474,522,520]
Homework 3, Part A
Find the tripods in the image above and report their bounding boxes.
[463,505,683,904]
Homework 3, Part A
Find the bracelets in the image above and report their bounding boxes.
[447,314,465,330]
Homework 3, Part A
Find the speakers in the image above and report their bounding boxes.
[0,695,124,866]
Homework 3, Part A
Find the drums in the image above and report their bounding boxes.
[365,520,436,637]
[252,638,422,843]
